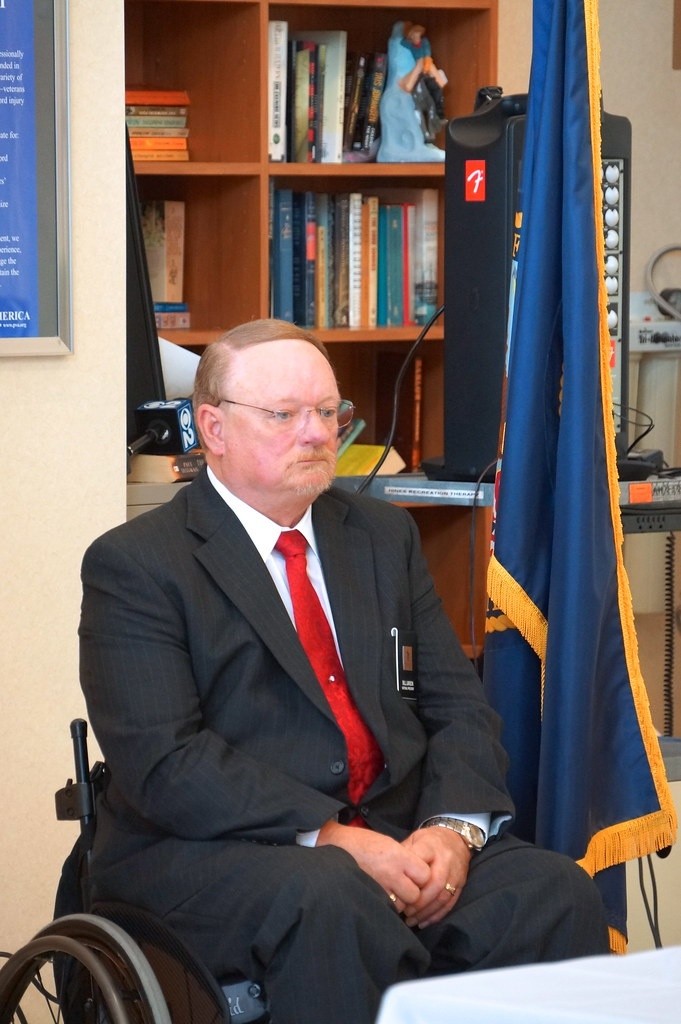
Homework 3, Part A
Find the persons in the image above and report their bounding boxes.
[77,318,608,1023]
[378,22,446,162]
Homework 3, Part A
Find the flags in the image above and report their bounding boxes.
[483,0,676,952]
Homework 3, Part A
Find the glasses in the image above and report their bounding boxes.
[216,399,356,428]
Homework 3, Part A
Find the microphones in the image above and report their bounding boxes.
[126,397,200,475]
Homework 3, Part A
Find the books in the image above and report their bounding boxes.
[331,474,495,506]
[268,182,439,328]
[126,86,192,161]
[141,198,185,303]
[378,352,422,475]
[268,20,389,163]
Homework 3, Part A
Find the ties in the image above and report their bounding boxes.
[275,528,385,829]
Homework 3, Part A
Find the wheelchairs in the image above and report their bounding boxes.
[1,717,274,1024]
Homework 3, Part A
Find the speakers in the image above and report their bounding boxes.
[423,91,632,480]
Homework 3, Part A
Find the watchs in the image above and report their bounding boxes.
[425,818,484,851]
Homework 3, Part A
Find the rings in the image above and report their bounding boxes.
[389,893,397,902]
[444,882,457,896]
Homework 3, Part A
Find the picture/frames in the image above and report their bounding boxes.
[0,0,75,358]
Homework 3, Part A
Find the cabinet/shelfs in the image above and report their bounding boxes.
[120,0,501,669]
